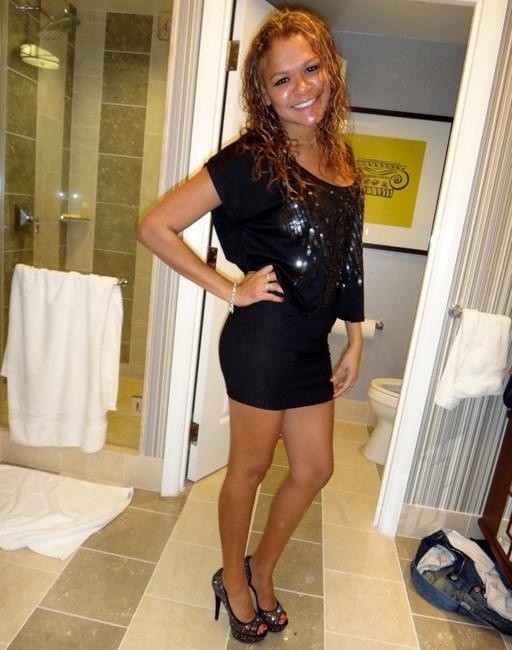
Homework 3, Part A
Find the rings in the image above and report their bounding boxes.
[266,274,270,282]
[266,283,269,292]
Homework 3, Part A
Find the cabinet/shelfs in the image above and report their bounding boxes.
[478,411,512,589]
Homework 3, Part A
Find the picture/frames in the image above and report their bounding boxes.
[330,107,454,256]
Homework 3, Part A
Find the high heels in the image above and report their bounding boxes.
[243,556,288,632]
[211,566,267,644]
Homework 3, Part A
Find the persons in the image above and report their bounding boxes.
[137,7,367,644]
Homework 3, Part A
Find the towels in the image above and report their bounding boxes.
[433,308,512,411]
[0,263,124,454]
[0,464,134,561]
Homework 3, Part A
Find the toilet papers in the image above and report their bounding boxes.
[330,319,378,341]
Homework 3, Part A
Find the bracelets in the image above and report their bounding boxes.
[229,282,238,314]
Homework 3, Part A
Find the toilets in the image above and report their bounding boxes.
[364,378,402,466]
